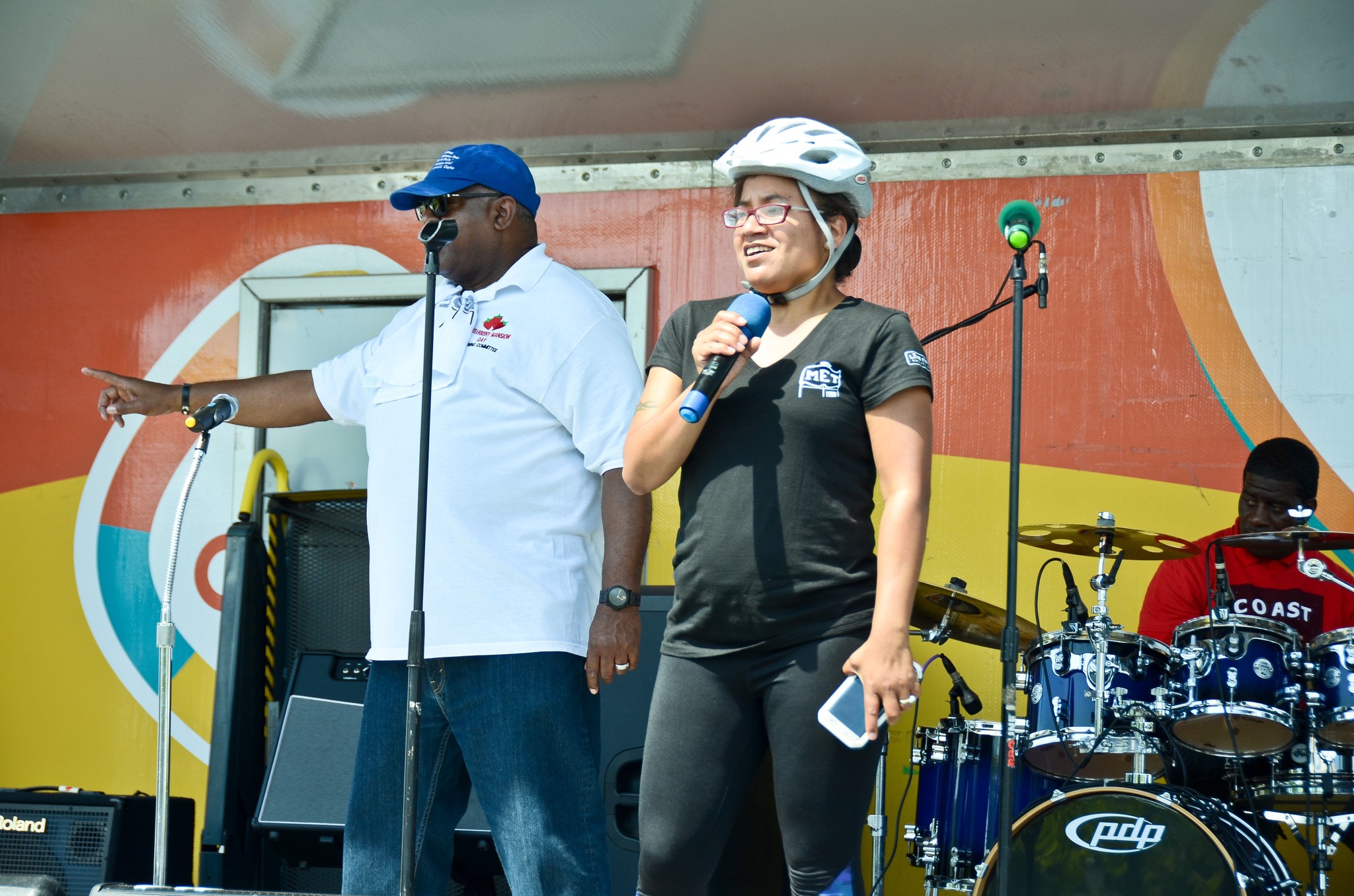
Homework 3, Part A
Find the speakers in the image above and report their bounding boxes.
[0,787,194,896]
[196,492,682,896]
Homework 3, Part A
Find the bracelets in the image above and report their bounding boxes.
[180,383,192,415]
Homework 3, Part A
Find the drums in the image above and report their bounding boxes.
[1307,627,1354,751]
[922,718,1074,892]
[971,773,1303,896]
[1173,614,1302,758]
[1021,626,1170,784]
[1241,726,1353,824]
[903,726,950,870]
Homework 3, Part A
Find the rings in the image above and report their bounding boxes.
[614,662,629,670]
[899,695,915,706]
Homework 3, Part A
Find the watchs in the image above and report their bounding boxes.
[597,584,640,609]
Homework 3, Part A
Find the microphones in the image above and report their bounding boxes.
[1037,241,1048,308]
[999,200,1041,251]
[1151,713,1186,787]
[1061,563,1088,624]
[1215,542,1235,614]
[679,293,772,423]
[939,655,982,715]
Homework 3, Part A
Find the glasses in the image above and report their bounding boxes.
[414,193,506,222]
[721,204,837,228]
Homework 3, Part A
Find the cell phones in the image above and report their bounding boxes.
[818,659,923,748]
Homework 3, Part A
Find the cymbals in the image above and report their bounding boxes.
[1214,524,1353,551]
[909,577,1049,653]
[1014,511,1200,560]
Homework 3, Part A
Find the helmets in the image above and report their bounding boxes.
[712,117,873,218]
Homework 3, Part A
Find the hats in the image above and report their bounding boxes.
[389,144,540,219]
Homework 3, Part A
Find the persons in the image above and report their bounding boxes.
[622,117,937,896]
[1132,438,1354,845]
[80,145,653,896]
